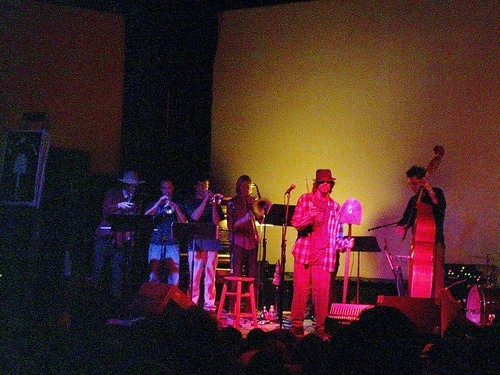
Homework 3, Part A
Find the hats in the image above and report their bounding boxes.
[120,171,141,185]
[314,169,336,181]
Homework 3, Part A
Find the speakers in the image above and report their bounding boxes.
[137,282,198,313]
[376,295,441,341]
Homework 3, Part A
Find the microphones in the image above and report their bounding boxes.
[285,184,296,195]
[263,206,267,217]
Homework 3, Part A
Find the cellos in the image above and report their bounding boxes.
[397,144,445,298]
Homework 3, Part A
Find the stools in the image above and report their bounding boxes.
[216,277,258,331]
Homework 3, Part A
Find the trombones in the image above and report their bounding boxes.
[242,182,273,244]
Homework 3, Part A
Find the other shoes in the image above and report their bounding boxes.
[314,330,332,342]
[203,306,216,313]
[289,324,304,337]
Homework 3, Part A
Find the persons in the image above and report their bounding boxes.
[1,293,500,375]
[92,170,272,316]
[395,166,447,305]
[291,170,354,340]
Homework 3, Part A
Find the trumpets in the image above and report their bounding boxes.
[163,191,174,215]
[204,190,223,205]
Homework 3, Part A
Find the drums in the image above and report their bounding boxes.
[464,283,500,330]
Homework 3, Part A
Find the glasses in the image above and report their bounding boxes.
[406,181,419,186]
